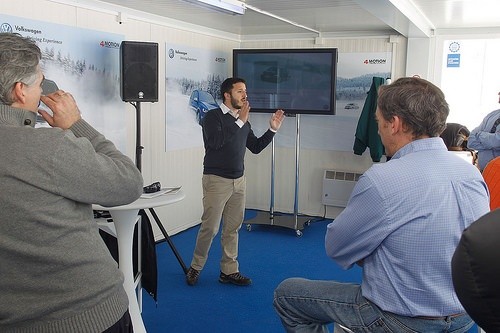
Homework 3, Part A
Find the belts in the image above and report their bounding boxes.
[415,312,463,321]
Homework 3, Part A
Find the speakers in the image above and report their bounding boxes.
[119,41,158,103]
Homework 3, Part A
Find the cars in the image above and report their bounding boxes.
[189,90,221,123]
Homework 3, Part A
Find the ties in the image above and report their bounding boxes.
[491,118,500,133]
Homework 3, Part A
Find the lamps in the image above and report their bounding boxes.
[178,0,245,16]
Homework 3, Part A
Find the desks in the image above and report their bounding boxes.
[92,188,186,333]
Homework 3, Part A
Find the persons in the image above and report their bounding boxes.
[451,207,500,333]
[466,91,500,174]
[0,31,142,333]
[274,76,490,333]
[188,78,285,286]
[441,122,470,150]
[482,157,500,212]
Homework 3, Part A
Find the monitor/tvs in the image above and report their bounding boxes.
[233,47,339,115]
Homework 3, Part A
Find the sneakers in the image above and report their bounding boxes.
[219,272,252,285]
[186,267,200,286]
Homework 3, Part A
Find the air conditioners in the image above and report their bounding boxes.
[322,169,364,207]
